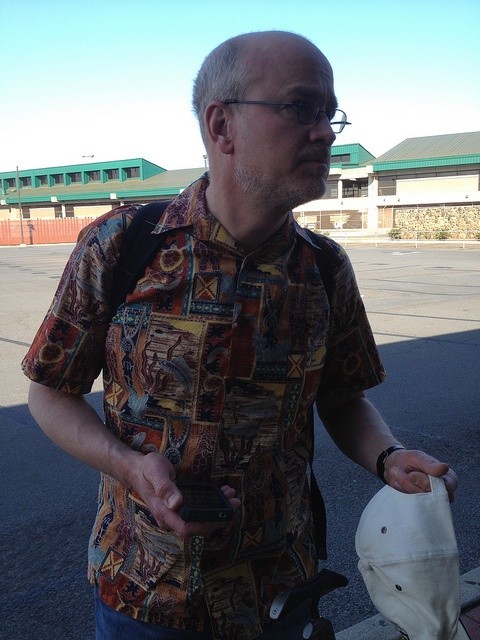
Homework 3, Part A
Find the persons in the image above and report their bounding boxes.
[22,31,457,640]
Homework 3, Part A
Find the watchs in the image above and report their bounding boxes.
[376,445,406,485]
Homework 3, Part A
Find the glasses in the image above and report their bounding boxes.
[223,97,352,135]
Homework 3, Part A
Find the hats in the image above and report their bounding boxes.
[354,473,471,640]
